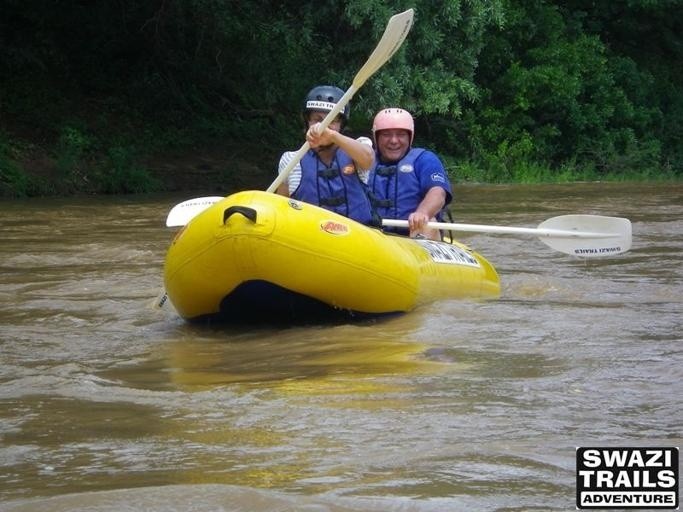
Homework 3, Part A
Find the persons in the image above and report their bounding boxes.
[364,107,453,243]
[276,85,378,227]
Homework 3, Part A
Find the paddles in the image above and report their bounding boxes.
[151,8,416,311]
[166,194,632,260]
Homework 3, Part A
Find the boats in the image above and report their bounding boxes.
[164,187,500,335]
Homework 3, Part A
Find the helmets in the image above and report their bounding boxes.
[303,85,350,124]
[372,107,415,152]
